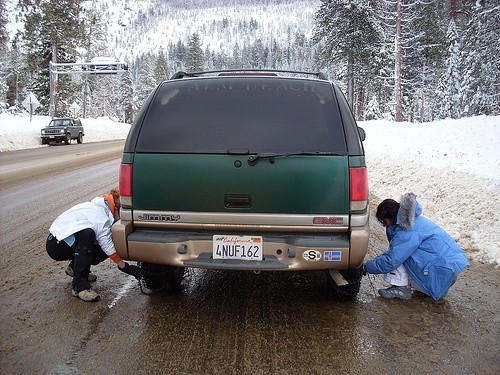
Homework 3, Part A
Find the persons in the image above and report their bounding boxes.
[358,192,470,302]
[46,188,143,302]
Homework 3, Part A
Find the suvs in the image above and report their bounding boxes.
[109,67,370,302]
[40,117,85,146]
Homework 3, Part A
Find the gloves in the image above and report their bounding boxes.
[118,262,144,280]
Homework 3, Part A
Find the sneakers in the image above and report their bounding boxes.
[65,264,97,282]
[378,284,412,300]
[72,289,100,302]
